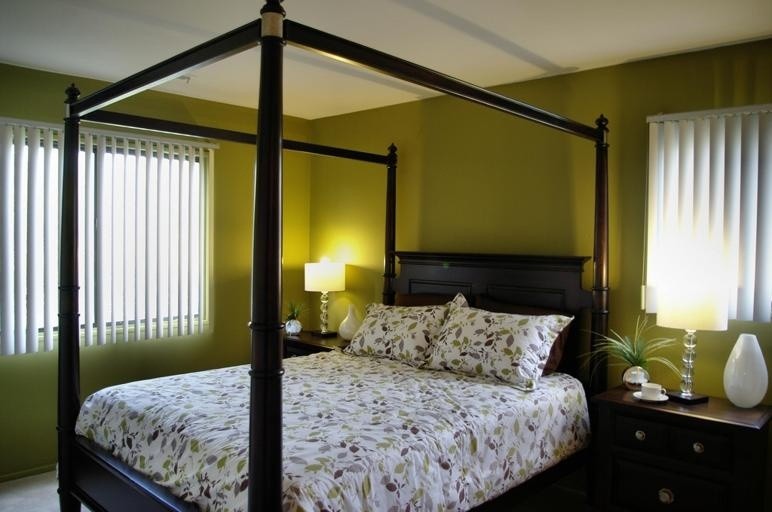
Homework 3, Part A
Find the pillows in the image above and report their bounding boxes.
[418,301,575,392]
[341,292,470,369]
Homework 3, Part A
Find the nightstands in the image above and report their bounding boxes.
[590,383,772,512]
[283,331,350,359]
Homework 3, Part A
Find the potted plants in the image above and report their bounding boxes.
[283,302,307,336]
[576,311,681,391]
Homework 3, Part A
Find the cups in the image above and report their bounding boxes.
[640,382,666,399]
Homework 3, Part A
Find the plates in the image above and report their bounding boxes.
[632,391,670,402]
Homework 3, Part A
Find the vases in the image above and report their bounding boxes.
[338,305,360,341]
[723,334,769,409]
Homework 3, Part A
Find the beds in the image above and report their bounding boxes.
[55,248,609,511]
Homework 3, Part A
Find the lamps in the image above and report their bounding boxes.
[655,280,729,405]
[304,263,346,339]
